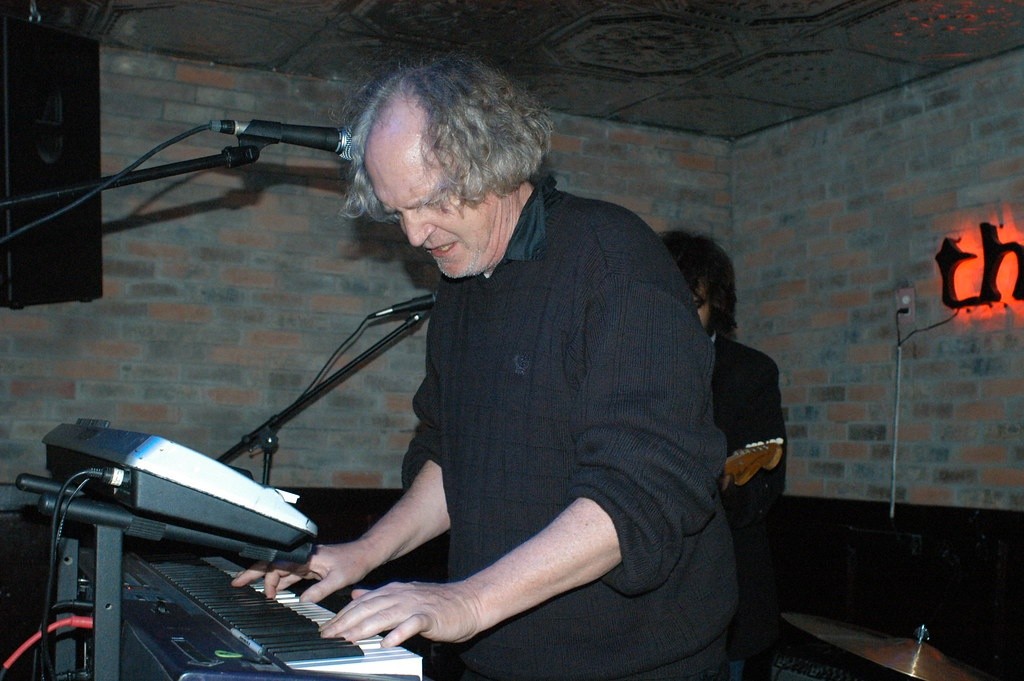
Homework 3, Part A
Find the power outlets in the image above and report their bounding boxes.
[899,288,915,324]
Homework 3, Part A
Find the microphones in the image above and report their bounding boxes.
[367,291,439,320]
[209,118,355,162]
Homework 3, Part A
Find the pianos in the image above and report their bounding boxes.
[76,541,426,681]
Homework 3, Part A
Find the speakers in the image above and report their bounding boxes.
[0,15,102,312]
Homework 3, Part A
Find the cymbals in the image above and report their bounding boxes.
[774,608,1002,680]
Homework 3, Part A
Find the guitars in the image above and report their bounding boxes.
[716,434,786,493]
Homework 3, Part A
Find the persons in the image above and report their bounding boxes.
[650,229,786,681]
[230,51,741,681]
